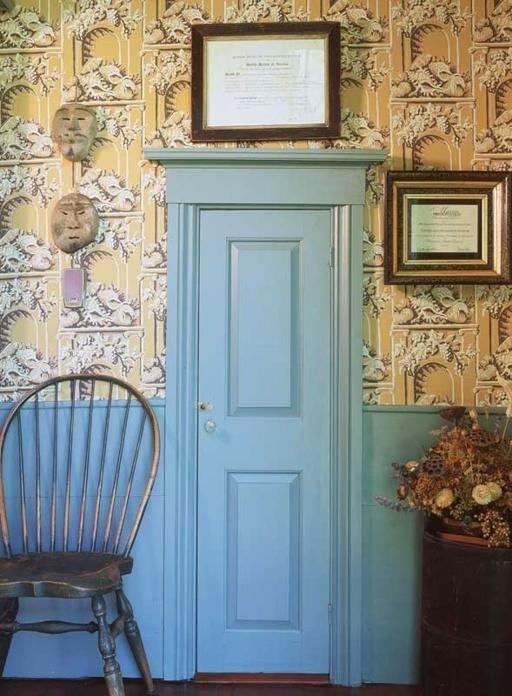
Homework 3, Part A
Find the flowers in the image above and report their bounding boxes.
[371,399,511,549]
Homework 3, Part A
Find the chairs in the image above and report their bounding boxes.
[0,372,161,696]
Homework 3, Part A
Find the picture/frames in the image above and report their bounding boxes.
[382,169,512,286]
[190,22,342,143]
